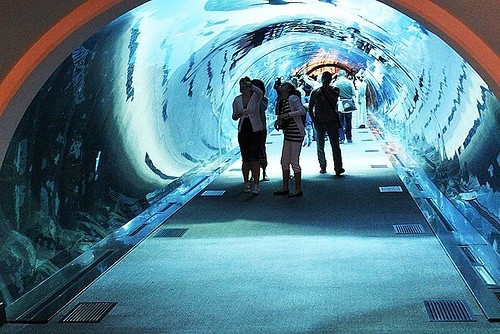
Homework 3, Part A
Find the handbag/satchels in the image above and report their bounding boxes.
[337,98,357,113]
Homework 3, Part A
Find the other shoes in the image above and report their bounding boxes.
[244,185,251,193]
[336,169,345,175]
[252,185,260,194]
[320,168,327,174]
[249,177,254,182]
[340,140,345,144]
[288,191,304,197]
[262,174,270,181]
[273,189,289,196]
[347,139,352,143]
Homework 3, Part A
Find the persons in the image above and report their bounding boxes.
[273,81,306,197]
[274,74,306,178]
[305,70,346,175]
[273,67,371,160]
[231,76,264,195]
[247,78,271,181]
